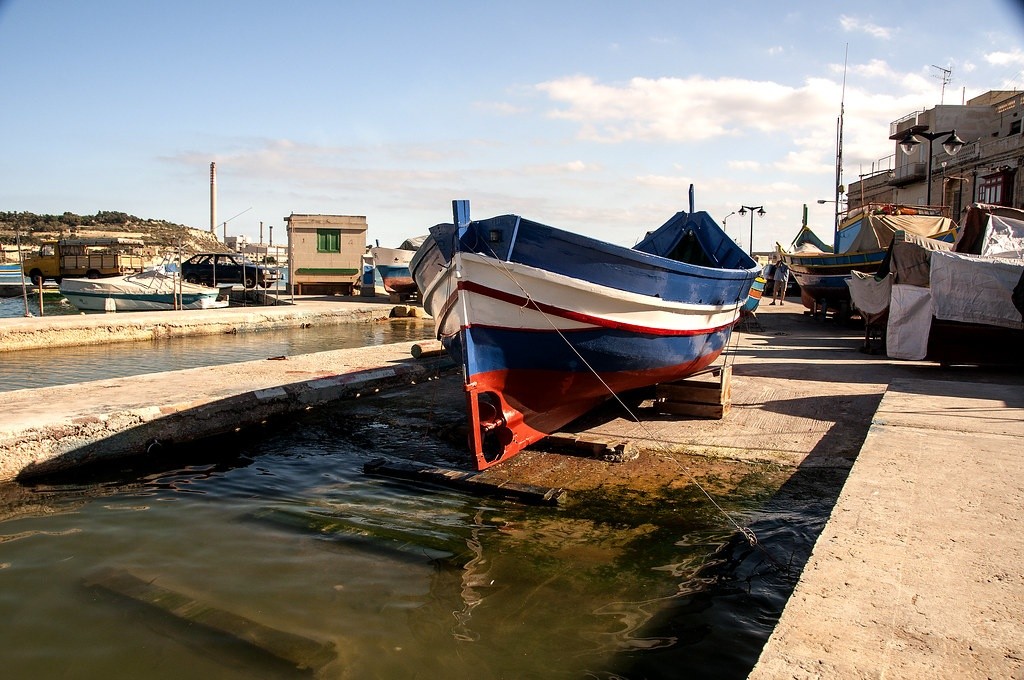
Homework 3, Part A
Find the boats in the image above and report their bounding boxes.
[775,65,962,325]
[59,270,221,311]
[370,235,432,292]
[407,183,763,473]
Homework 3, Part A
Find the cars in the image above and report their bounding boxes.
[179,252,283,289]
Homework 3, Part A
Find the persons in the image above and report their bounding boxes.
[769,259,790,305]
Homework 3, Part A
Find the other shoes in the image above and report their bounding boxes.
[780,302,784,306]
[769,302,775,305]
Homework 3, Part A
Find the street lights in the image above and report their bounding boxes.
[888,124,966,209]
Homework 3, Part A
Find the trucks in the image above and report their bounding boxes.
[21,236,145,286]
